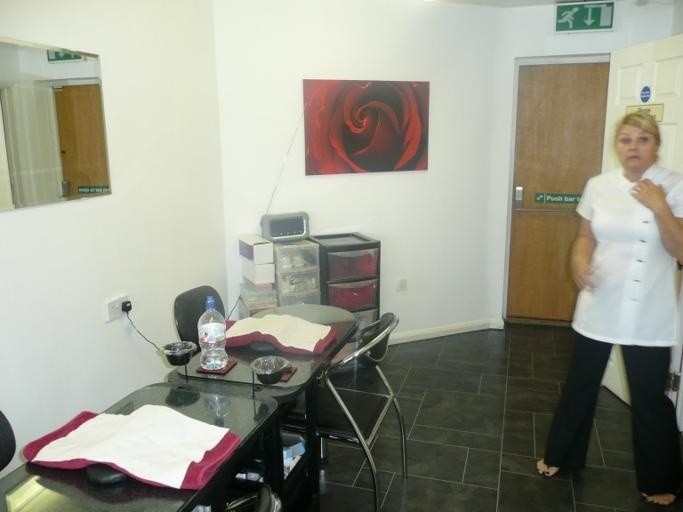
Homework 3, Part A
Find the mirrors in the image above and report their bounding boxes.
[0,38,113,214]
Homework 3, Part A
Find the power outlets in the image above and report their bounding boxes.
[103,294,129,322]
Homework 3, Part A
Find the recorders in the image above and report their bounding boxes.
[261,211,310,240]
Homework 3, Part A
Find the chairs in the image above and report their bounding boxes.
[279,311,406,510]
[173,286,239,362]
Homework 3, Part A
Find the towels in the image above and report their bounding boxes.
[24,404,240,490]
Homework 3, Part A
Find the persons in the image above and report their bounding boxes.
[532,110,683,506]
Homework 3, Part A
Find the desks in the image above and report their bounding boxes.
[0,386,279,511]
[176,301,360,495]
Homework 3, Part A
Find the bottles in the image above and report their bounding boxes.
[204,392,232,428]
[197,294,229,371]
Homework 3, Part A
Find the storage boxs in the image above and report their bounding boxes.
[239,236,321,318]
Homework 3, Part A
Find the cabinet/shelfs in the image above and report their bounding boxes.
[307,233,380,326]
